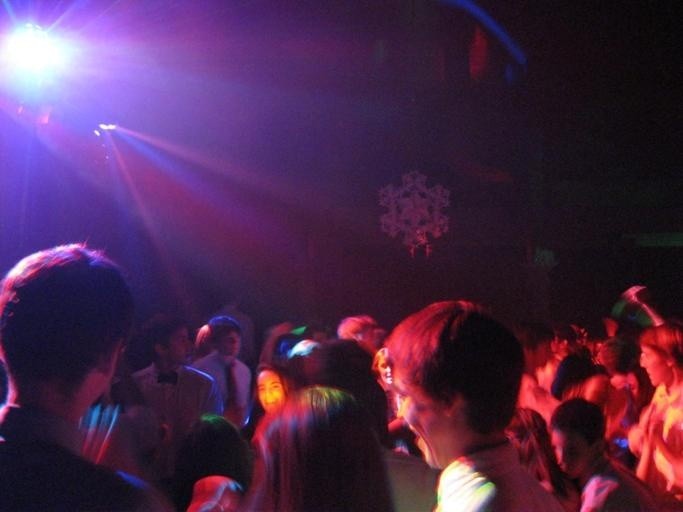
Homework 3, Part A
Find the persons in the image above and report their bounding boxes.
[0,239,174,510]
[75,300,562,511]
[503,282,682,512]
